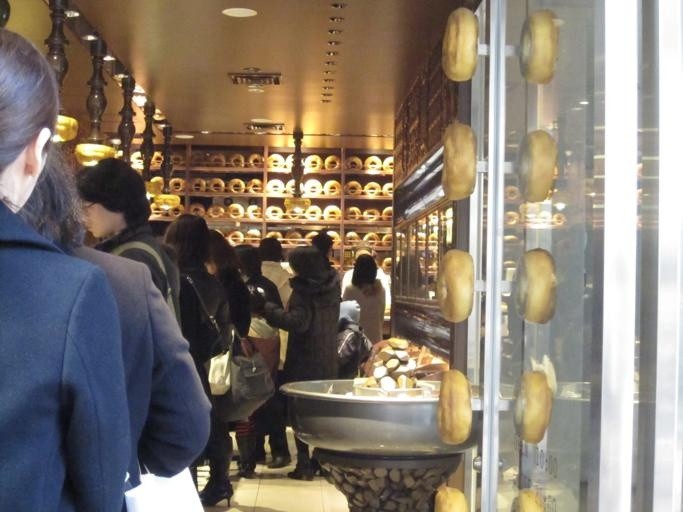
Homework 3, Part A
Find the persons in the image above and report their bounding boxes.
[259,237,292,362]
[337,300,369,380]
[76,157,175,305]
[23,149,211,492]
[0,19,131,511]
[341,247,392,305]
[160,214,233,507]
[252,246,341,480]
[232,244,291,468]
[202,229,255,479]
[342,254,385,344]
[311,230,334,258]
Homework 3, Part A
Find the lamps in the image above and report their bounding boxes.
[44,0,79,142]
[74,37,116,167]
[141,106,162,198]
[154,126,180,208]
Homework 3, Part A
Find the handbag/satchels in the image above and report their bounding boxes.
[185,278,230,396]
[215,323,275,422]
[123,463,205,512]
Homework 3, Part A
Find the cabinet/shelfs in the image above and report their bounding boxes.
[394,200,453,305]
[188,144,264,250]
[118,139,188,223]
[266,144,342,274]
[343,151,394,271]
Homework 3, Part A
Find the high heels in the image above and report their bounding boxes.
[296,459,328,476]
[287,462,313,481]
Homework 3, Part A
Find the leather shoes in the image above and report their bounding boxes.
[268,454,291,468]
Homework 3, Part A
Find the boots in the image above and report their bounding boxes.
[229,436,257,481]
[199,437,234,508]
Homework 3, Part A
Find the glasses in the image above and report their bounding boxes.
[83,201,96,209]
